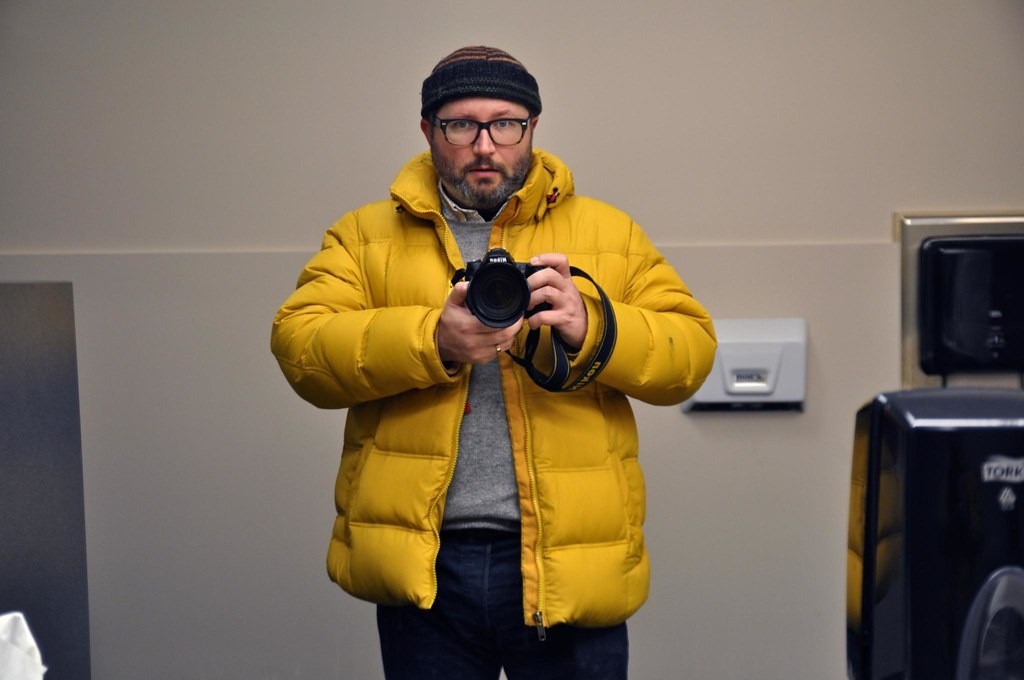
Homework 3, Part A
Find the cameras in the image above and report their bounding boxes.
[460,248,554,328]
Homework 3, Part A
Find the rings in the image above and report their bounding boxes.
[496,344,501,355]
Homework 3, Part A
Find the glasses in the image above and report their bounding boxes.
[426,110,534,146]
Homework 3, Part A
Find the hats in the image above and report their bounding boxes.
[421,47,543,119]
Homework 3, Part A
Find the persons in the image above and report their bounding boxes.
[270,44,718,680]
